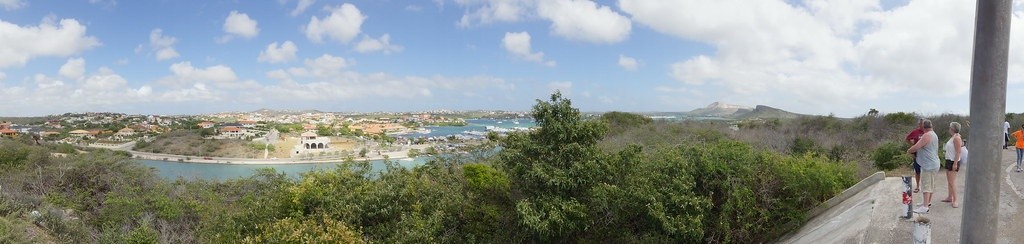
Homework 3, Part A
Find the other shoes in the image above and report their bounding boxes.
[913,206,930,213]
[917,202,931,207]
[913,189,919,193]
[1020,168,1023,171]
[940,198,952,202]
[1017,168,1020,172]
[952,201,958,208]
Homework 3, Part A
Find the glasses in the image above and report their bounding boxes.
[1022,127,1024,129]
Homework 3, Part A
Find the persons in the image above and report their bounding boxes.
[907,119,940,214]
[1004,120,1011,149]
[1011,124,1024,172]
[941,121,963,209]
[905,117,927,192]
